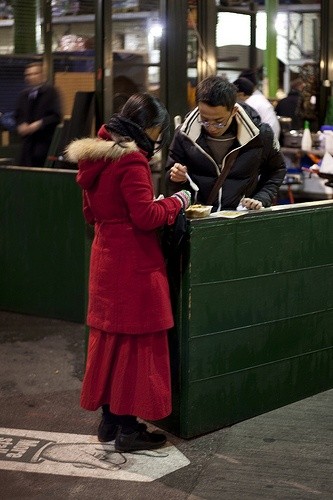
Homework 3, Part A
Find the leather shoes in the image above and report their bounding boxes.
[114,432,168,451]
[96,412,160,438]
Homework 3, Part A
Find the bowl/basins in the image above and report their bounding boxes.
[184,205,213,218]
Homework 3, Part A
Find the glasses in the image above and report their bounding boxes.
[197,107,233,129]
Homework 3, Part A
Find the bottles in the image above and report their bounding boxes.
[301,121,313,150]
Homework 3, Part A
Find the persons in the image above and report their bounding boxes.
[63,91,192,454]
[162,75,287,210]
[238,69,281,153]
[13,62,62,168]
[110,49,322,127]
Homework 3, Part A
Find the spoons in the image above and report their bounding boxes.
[184,172,199,191]
